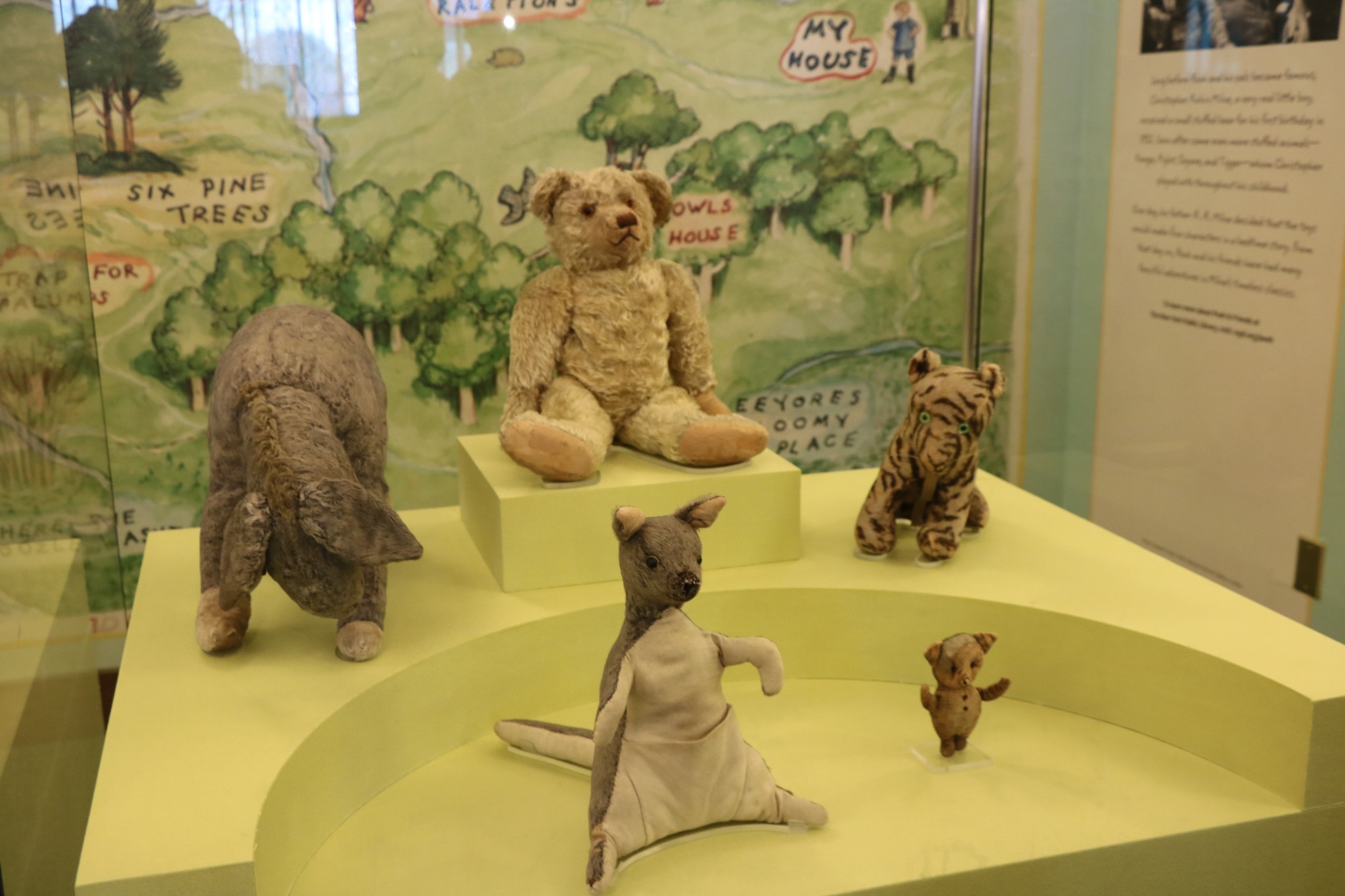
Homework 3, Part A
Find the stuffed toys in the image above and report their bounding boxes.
[495,495,828,891]
[921,633,1010,757]
[194,305,424,660]
[855,348,1007,560]
[499,165,769,481]
